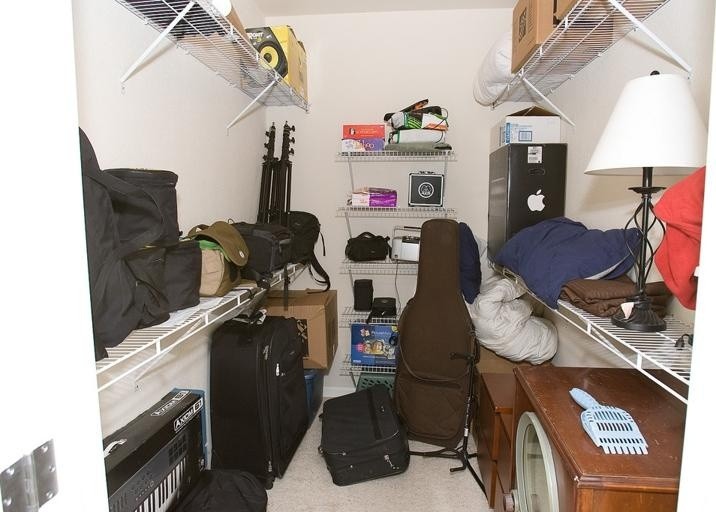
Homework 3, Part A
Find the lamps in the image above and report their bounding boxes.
[584,70,709,331]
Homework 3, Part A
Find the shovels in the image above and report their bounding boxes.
[569,387,649,457]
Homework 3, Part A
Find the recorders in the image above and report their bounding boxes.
[391,226,421,262]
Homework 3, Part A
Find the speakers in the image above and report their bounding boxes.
[241,26,288,85]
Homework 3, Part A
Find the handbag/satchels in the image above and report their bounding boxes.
[228,217,295,274]
[80,127,204,362]
[182,220,248,298]
[345,232,389,262]
[287,212,320,263]
[170,467,267,512]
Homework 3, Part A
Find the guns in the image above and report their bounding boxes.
[256,121,295,229]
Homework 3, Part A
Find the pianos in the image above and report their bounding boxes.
[108,425,195,512]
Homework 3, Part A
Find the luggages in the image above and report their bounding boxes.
[210,308,309,488]
[317,385,410,486]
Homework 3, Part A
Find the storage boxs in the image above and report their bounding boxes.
[490,106,561,154]
[241,25,308,105]
[511,0,612,72]
[265,288,338,369]
[556,1,666,21]
[350,319,398,366]
[103,390,209,512]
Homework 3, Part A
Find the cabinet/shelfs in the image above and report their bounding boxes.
[472,366,688,512]
[487,1,716,406]
[333,144,457,380]
